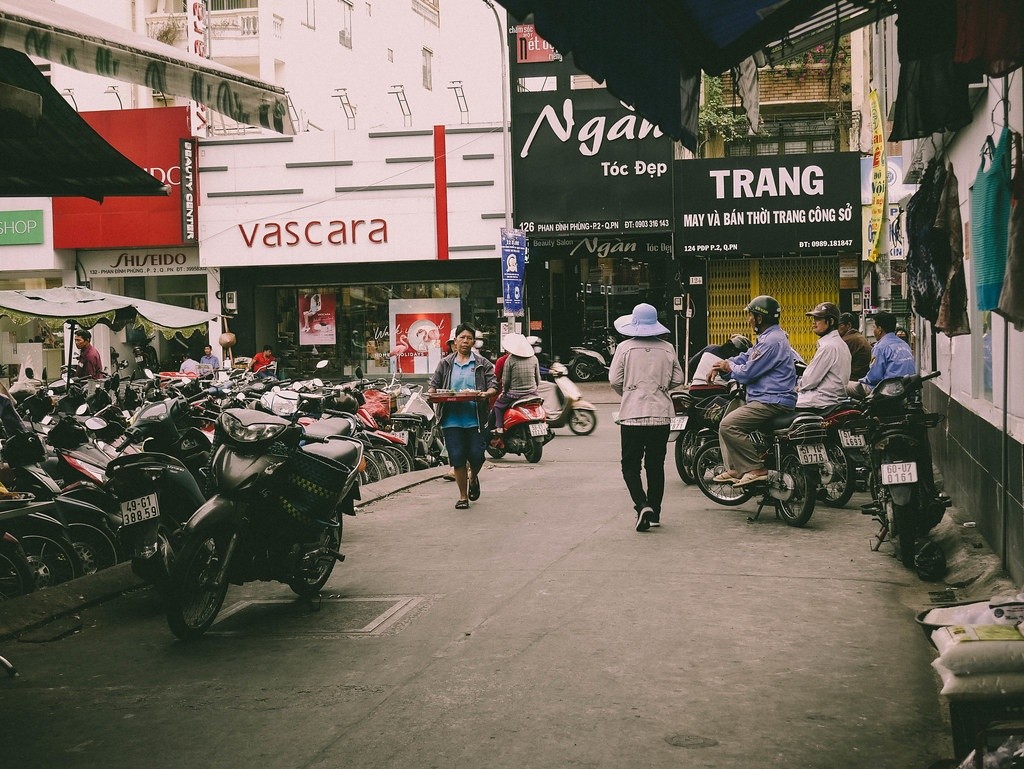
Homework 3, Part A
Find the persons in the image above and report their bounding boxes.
[608,303,685,530]
[247,345,276,379]
[838,313,871,381]
[302,295,320,333]
[179,353,201,374]
[846,311,917,400]
[199,345,220,389]
[427,321,554,509]
[794,302,852,413]
[781,330,804,363]
[706,295,796,487]
[689,334,752,385]
[72,330,102,379]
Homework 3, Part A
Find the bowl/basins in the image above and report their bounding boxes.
[914,599,991,651]
[436,389,449,393]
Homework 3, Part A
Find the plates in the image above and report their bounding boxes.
[431,393,455,397]
[451,391,481,396]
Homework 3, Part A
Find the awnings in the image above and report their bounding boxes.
[497,0,897,157]
[0,0,296,200]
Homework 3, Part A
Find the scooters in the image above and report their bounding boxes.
[843,371,953,570]
[486,392,550,463]
[546,354,598,436]
[0,335,453,601]
[670,334,875,526]
[568,326,620,383]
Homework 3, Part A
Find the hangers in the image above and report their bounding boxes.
[927,129,952,166]
[979,101,1011,155]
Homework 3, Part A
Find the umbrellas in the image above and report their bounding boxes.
[0,284,222,396]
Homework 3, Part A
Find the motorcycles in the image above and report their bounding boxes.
[163,394,371,642]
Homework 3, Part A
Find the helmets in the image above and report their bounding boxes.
[727,335,753,352]
[743,295,781,321]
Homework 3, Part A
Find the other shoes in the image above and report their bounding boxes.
[635,503,654,532]
[443,473,456,481]
[489,429,505,434]
[455,499,469,508]
[468,476,481,502]
[649,517,660,527]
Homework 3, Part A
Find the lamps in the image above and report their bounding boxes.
[62,89,79,112]
[389,85,411,117]
[104,85,123,110]
[332,88,355,119]
[448,81,468,112]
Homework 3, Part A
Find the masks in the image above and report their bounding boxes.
[533,347,542,353]
[475,340,484,348]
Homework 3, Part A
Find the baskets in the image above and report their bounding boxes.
[396,383,423,410]
[694,394,731,427]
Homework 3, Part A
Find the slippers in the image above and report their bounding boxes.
[732,473,769,488]
[712,472,741,482]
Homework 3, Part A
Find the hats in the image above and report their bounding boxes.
[525,335,542,345]
[836,312,857,326]
[502,332,535,357]
[614,302,671,336]
[446,328,457,345]
[475,330,484,339]
[805,302,840,320]
